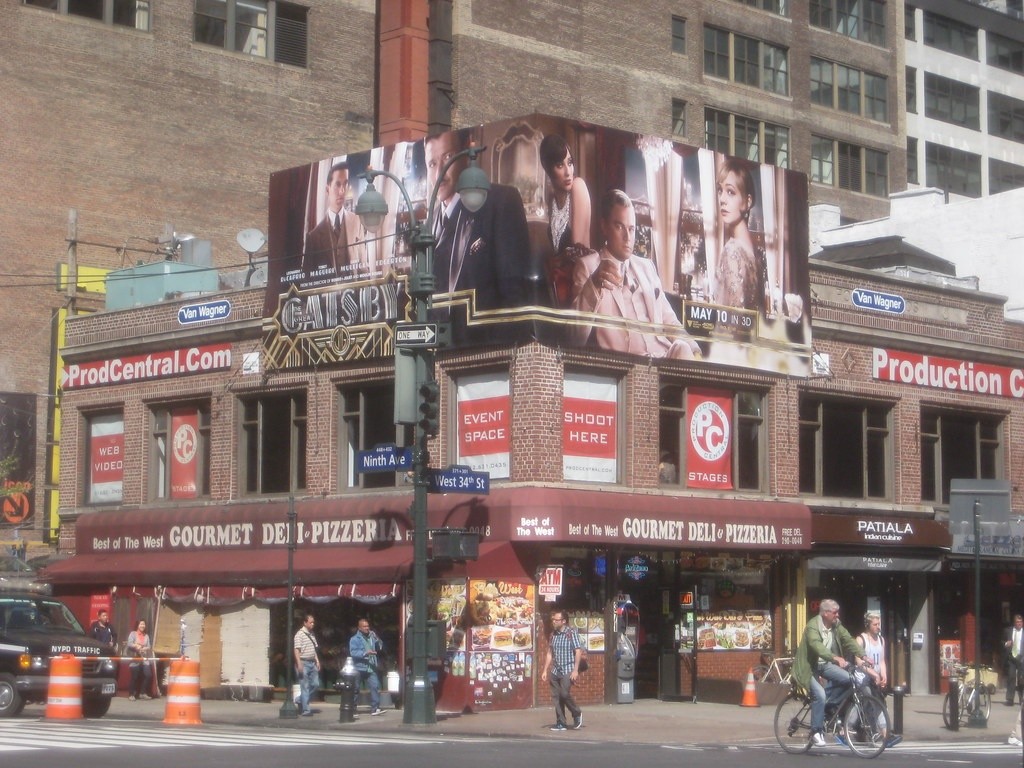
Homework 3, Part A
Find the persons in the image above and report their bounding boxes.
[568,189,702,362]
[294,614,320,716]
[1001,614,1024,706]
[714,162,767,314]
[629,614,647,649]
[790,600,875,747]
[125,618,153,702]
[349,618,388,719]
[542,612,583,731]
[835,611,903,748]
[534,134,591,263]
[302,161,369,290]
[424,126,535,345]
[90,609,118,654]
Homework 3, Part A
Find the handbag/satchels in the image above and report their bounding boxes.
[123,631,136,657]
[578,657,588,672]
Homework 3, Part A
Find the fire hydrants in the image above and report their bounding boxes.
[332,657,358,722]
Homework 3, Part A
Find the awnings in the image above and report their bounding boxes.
[811,512,953,547]
[42,541,516,604]
[75,487,811,549]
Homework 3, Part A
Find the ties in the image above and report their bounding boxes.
[620,261,631,292]
[333,214,341,241]
[1012,630,1019,658]
[441,214,448,234]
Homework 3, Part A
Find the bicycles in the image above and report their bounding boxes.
[773,660,891,759]
[754,645,810,702]
[943,658,990,728]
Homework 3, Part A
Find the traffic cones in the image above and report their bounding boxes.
[739,666,761,707]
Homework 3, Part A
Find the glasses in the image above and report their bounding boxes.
[551,617,564,622]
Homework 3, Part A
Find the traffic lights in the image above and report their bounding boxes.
[419,380,440,437]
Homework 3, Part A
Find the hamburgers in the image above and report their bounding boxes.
[493,630,513,646]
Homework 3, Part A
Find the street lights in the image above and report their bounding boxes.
[354,145,491,729]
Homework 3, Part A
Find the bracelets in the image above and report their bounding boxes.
[316,661,319,663]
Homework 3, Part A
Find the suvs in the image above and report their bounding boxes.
[0,593,121,719]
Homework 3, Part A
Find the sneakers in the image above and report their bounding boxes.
[353,709,360,719]
[551,723,568,731]
[834,731,849,748]
[884,734,903,748]
[370,707,386,715]
[573,712,582,729]
[810,733,826,747]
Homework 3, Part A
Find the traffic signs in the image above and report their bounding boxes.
[393,321,439,349]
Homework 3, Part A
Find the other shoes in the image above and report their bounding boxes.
[303,712,313,717]
[129,694,135,701]
[139,693,153,699]
[1007,698,1013,705]
[297,701,304,715]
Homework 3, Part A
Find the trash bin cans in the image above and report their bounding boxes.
[618,659,635,704]
